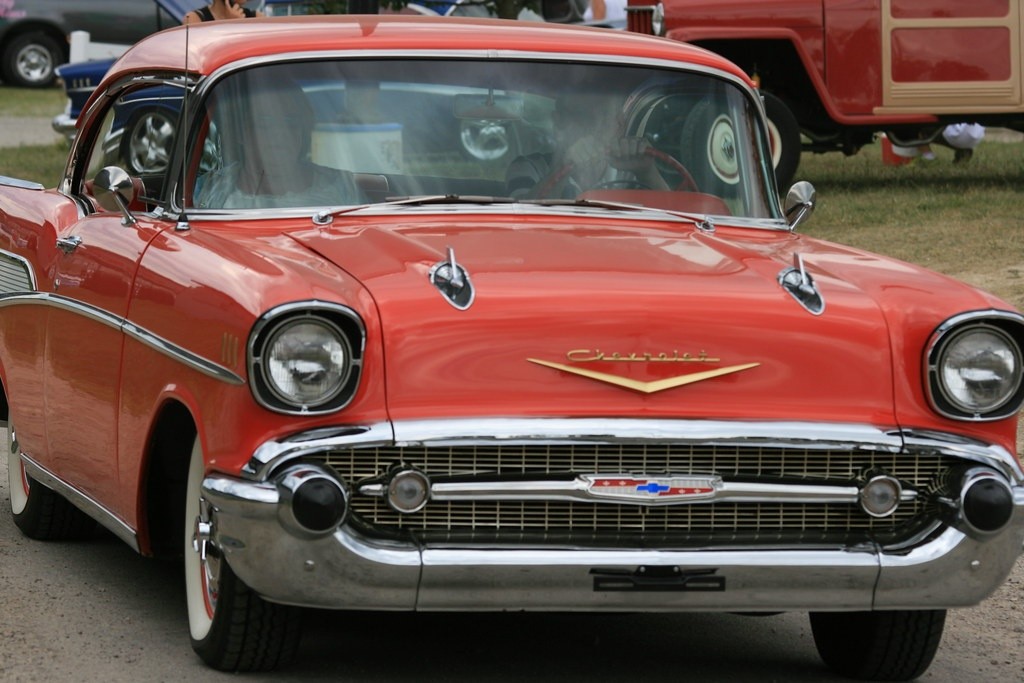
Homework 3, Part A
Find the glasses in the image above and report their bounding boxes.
[256,114,302,131]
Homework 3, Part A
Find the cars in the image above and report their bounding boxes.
[617,1,1024,196]
[0,2,235,88]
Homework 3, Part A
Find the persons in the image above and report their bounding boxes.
[191,76,371,208]
[506,81,670,199]
[181,0,265,25]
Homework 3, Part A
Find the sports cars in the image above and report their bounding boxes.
[0,9,1023,683]
